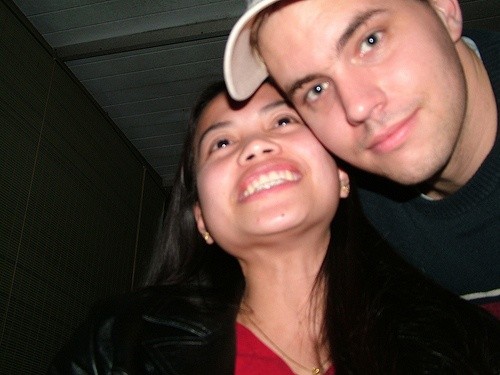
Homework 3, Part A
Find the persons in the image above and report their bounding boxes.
[44,79,499,374]
[222,0,499,320]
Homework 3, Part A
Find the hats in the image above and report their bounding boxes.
[223,0,281,101]
